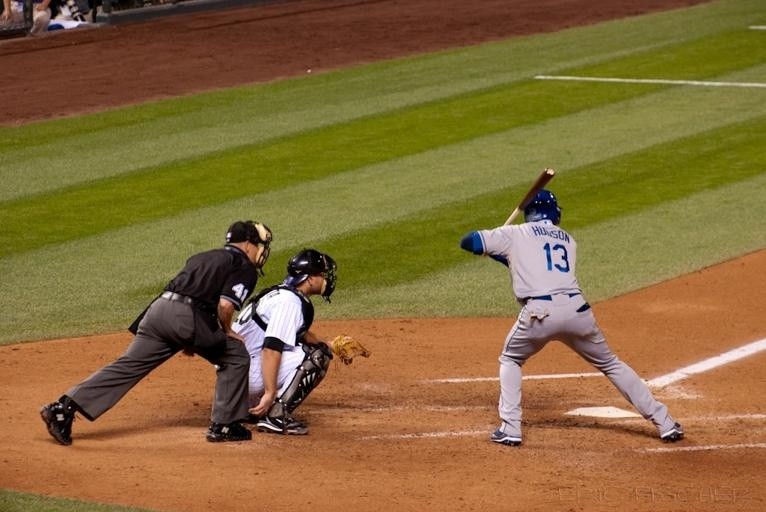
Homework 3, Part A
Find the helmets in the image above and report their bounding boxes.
[525,190,561,222]
[284,249,337,285]
[226,220,272,245]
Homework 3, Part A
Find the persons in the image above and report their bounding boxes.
[215,247,372,436]
[39,218,274,446]
[0,1,173,38]
[459,188,685,445]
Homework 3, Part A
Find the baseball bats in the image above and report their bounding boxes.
[504,166,556,226]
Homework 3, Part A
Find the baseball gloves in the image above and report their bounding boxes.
[333,334,371,367]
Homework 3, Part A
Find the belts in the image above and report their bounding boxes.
[532,292,579,300]
[161,291,192,303]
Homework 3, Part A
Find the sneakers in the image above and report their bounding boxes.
[490,431,521,444]
[661,423,684,439]
[256,413,308,434]
[41,401,72,445]
[207,423,251,442]
